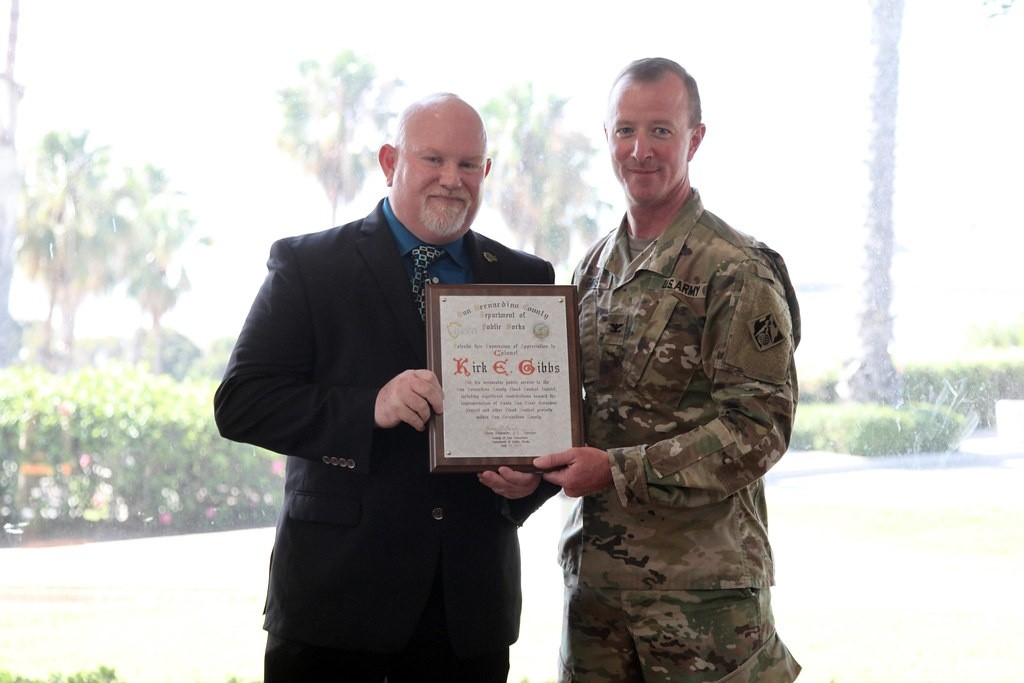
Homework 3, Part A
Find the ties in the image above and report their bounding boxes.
[409,245,446,322]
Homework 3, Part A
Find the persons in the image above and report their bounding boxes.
[534,59,802,683]
[214,95,564,683]
